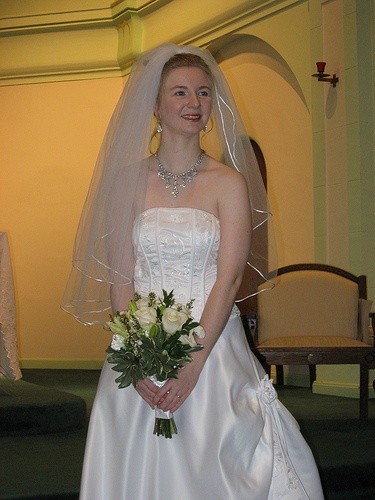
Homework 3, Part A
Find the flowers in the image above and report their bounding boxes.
[103,288,205,440]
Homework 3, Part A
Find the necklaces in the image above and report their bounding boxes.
[154,150,208,198]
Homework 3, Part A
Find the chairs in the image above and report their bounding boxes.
[251,262,374,421]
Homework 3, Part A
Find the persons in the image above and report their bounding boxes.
[103,53,254,500]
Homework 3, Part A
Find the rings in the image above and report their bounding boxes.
[176,394,182,398]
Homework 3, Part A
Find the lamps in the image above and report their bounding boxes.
[310,61,339,89]
